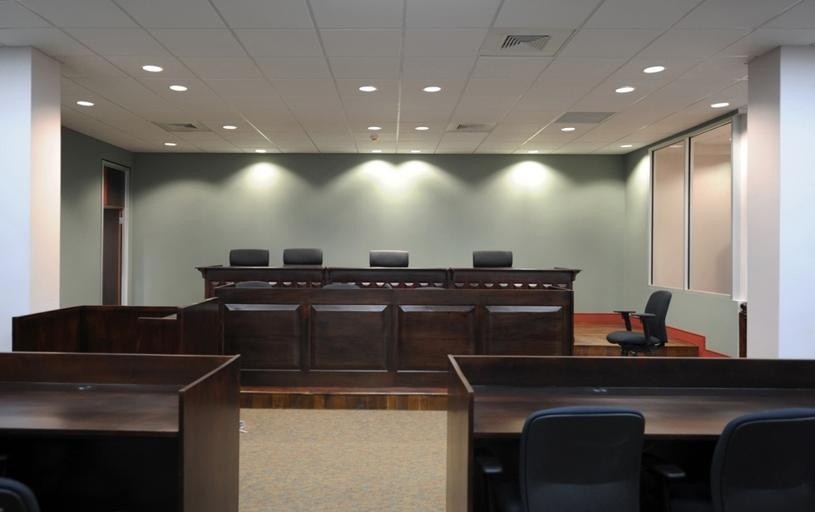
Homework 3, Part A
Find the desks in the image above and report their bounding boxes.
[0,346,242,511]
[443,351,815,512]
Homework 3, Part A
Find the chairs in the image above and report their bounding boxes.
[0,478,42,510]
[283,248,323,265]
[710,407,815,511]
[230,249,269,266]
[369,250,408,267]
[472,250,513,267]
[607,290,673,356]
[510,406,646,512]
